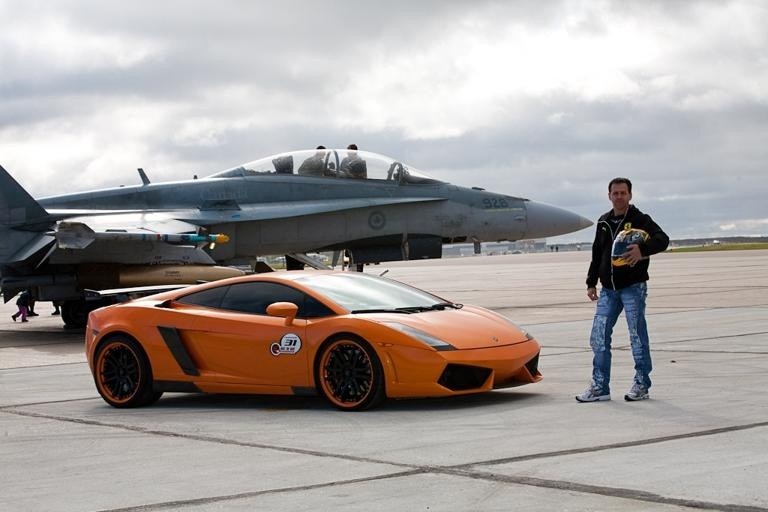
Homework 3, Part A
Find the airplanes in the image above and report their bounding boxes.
[1,148,594,334]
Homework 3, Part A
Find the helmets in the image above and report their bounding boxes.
[609,227,651,268]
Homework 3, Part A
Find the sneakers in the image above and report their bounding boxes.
[574,386,612,403]
[622,383,650,401]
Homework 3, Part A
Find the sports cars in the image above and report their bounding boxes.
[84,271,542,412]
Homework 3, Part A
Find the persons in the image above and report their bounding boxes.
[297,146,337,178]
[11,290,39,322]
[340,143,366,179]
[51,300,63,315]
[575,176,670,403]
